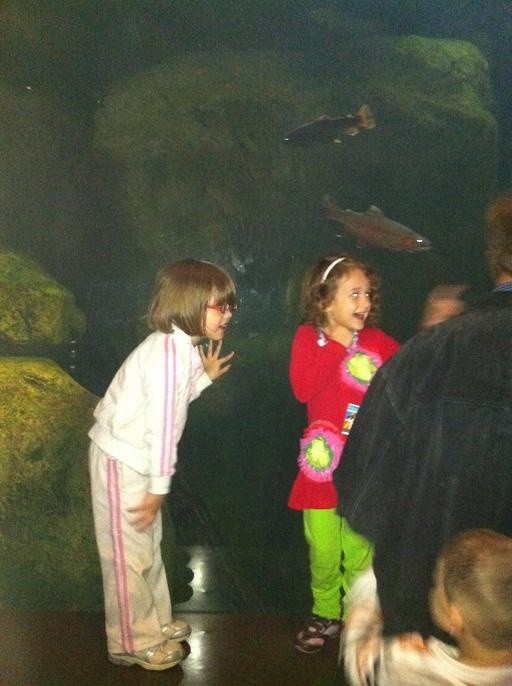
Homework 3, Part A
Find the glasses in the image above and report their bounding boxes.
[206,302,238,314]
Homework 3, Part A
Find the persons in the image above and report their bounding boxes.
[416,283,471,324]
[88,257,236,672]
[333,188,511,647]
[288,252,400,658]
[338,527,511,686]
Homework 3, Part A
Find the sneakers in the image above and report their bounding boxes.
[109,619,191,671]
[295,615,341,654]
